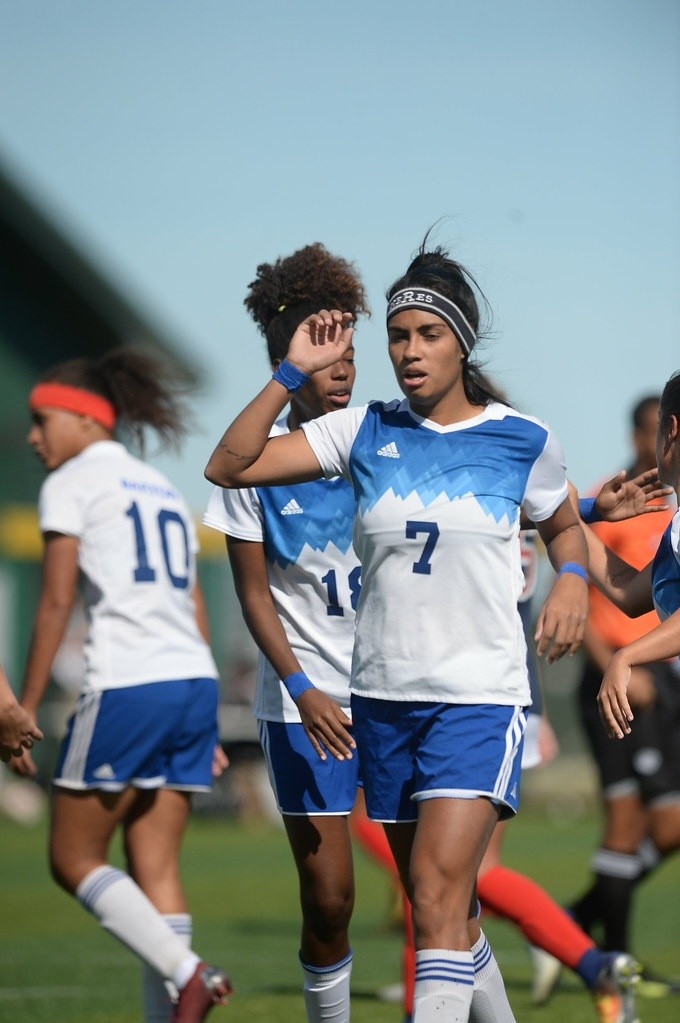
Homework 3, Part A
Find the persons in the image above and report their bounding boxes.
[0,667,44,766]
[204,212,680,1023]
[11,332,232,1023]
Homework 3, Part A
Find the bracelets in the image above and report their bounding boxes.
[272,360,308,395]
[561,560,590,586]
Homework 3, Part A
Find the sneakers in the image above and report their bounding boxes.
[163,962,233,1023]
[592,951,645,1023]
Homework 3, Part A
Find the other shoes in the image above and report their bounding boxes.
[526,943,560,1005]
